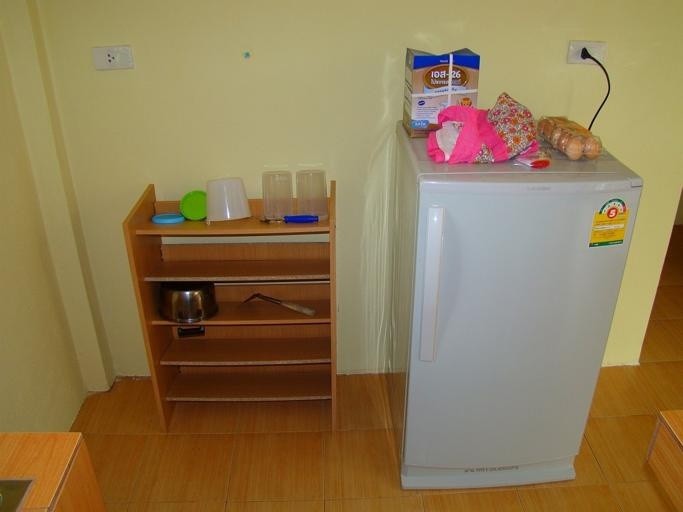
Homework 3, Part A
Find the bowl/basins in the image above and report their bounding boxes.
[159,282,221,326]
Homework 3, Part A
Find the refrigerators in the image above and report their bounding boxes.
[382,121,646,491]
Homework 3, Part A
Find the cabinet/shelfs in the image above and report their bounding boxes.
[0,430,103,511]
[120,180,343,437]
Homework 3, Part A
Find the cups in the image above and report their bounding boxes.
[296,168,328,223]
[261,170,293,222]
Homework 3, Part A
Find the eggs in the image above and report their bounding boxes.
[539,117,601,160]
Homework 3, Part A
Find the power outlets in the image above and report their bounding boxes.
[567,42,607,65]
[94,46,134,71]
